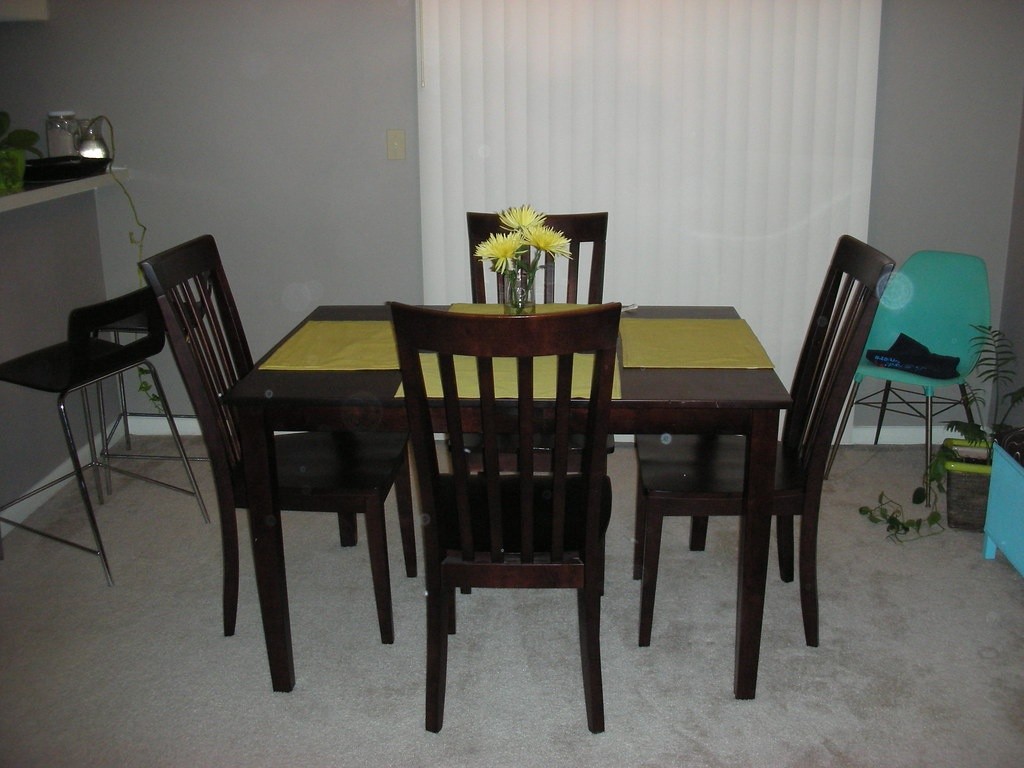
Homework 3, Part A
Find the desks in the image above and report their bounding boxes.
[218,304,791,701]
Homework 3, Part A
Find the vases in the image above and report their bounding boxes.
[78,116,109,159]
[502,273,537,315]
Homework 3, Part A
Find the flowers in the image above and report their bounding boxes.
[472,206,572,315]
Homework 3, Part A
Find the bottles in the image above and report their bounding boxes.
[77,118,110,159]
[46,110,82,163]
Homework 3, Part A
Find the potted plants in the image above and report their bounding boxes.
[0,108,44,195]
[858,326,1023,543]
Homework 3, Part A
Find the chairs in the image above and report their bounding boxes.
[631,234,896,648]
[138,235,417,645]
[384,300,623,735]
[824,250,993,508]
[0,281,212,589]
[444,210,616,596]
